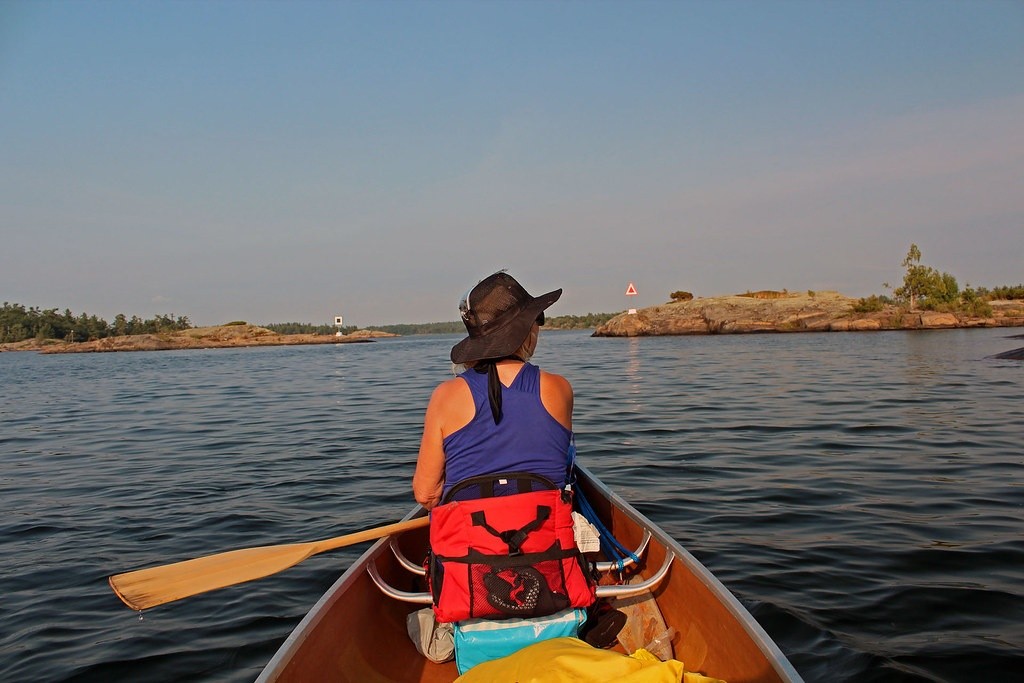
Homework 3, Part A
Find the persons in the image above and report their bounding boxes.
[408,273,575,587]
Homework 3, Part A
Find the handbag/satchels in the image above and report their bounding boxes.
[424,487,597,624]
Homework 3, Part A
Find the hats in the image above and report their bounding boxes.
[450,272,562,363]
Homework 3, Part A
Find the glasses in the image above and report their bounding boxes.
[535,312,545,326]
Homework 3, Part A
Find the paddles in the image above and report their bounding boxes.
[107,516,429,611]
